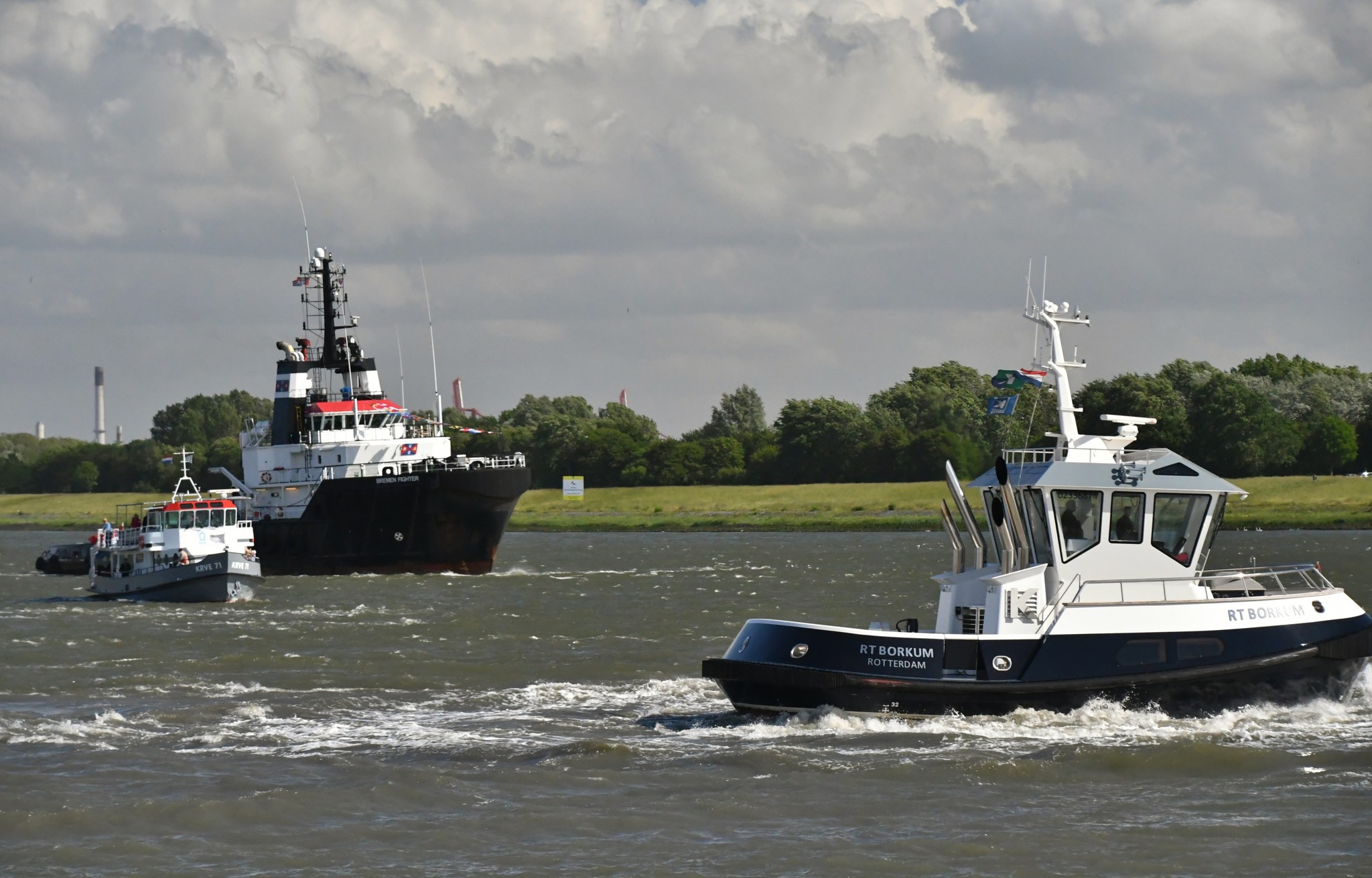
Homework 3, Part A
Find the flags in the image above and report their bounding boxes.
[336,277,344,285]
[181,454,193,464]
[386,406,496,435]
[1013,368,1047,389]
[986,394,1020,415]
[991,370,1026,393]
[292,277,310,286]
[162,453,173,463]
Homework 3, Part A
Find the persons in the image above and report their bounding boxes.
[173,549,190,567]
[119,558,132,577]
[1116,506,1137,542]
[1063,500,1084,541]
[97,555,117,578]
[131,513,142,537]
[211,512,219,527]
[187,515,205,528]
[325,420,332,430]
[118,522,126,545]
[142,512,154,532]
[102,518,113,549]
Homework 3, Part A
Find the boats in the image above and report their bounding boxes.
[35,179,532,576]
[85,445,266,604]
[701,255,1372,718]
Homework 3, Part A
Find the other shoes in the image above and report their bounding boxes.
[109,545,111,547]
[106,546,108,548]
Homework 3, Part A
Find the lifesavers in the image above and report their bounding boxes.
[278,507,283,517]
[262,473,272,483]
[139,536,145,549]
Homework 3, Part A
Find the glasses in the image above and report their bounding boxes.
[103,520,107,522]
